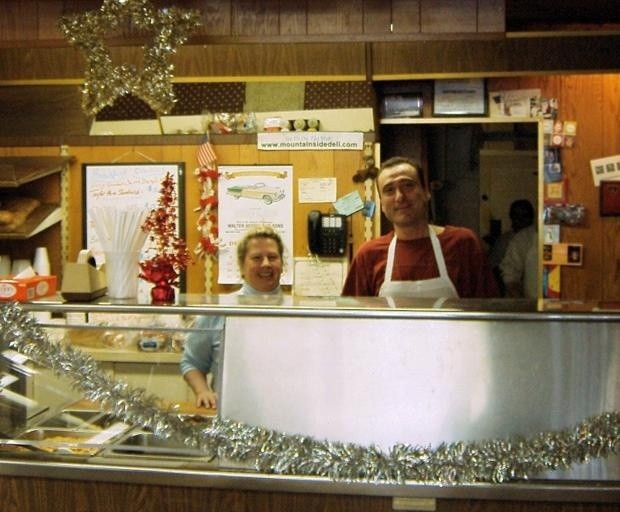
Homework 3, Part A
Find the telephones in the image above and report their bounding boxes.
[307,210,346,257]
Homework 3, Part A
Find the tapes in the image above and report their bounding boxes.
[77,248,97,269]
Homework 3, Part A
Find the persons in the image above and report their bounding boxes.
[340,157,502,299]
[499,224,537,298]
[180,226,284,410]
[492,199,534,295]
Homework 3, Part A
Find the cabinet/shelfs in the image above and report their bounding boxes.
[1,154,70,292]
[480,149,538,238]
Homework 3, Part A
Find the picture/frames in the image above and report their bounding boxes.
[383,92,423,118]
[432,79,488,117]
[83,163,184,299]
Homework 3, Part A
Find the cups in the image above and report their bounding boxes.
[0,246,52,320]
